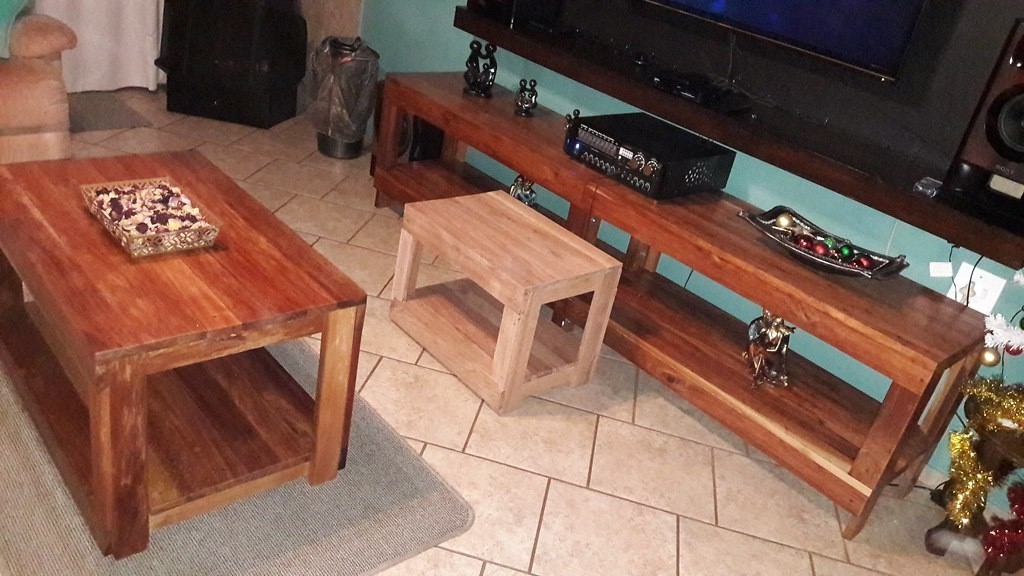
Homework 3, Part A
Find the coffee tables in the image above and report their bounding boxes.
[0,149,367,561]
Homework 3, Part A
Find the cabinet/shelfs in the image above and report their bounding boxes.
[374,71,987,541]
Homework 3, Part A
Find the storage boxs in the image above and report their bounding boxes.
[79,176,222,260]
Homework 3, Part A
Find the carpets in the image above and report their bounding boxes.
[0,281,473,576]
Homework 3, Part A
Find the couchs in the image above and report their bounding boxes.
[0,14,77,165]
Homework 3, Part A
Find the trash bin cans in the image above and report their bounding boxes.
[309,36,380,160]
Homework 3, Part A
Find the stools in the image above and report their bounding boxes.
[389,191,623,415]
[947,385,1023,523]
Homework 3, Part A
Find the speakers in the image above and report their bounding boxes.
[941,20,1024,231]
[151,2,309,129]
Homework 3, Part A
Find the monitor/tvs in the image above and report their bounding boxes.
[467,0,1024,194]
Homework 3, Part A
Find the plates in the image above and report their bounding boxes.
[737,205,911,280]
